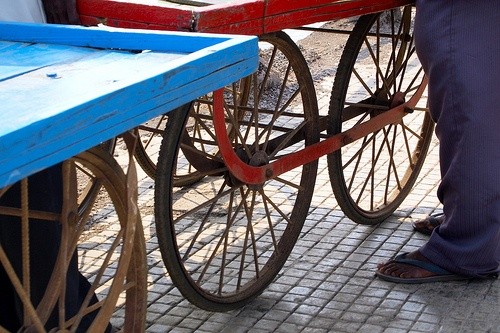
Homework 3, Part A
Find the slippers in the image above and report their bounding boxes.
[375,251,473,282]
[412,212,446,235]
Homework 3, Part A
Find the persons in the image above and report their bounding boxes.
[1,0,115,332]
[375,0,500,282]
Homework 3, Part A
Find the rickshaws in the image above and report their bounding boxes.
[0,0,451,333]
